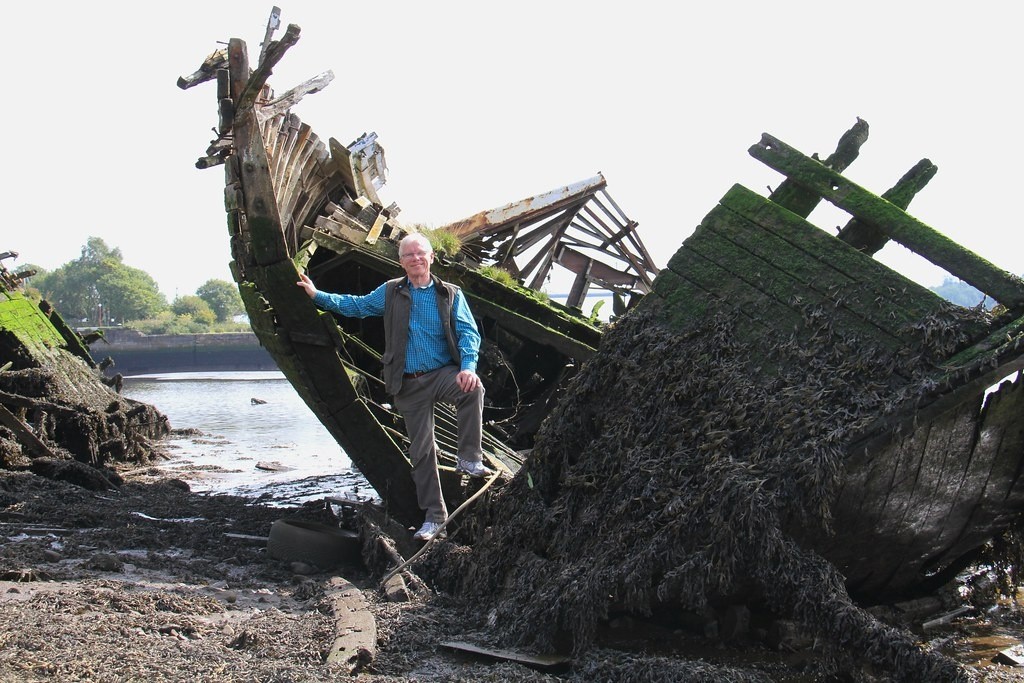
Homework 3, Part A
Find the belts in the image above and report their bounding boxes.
[403,371,427,379]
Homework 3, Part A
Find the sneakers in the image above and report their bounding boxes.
[414,521,447,541]
[456,456,492,476]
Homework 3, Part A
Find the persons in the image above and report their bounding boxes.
[296,233,492,540]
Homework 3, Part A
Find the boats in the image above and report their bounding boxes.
[177,5,1024,620]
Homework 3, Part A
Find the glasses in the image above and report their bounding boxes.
[400,251,430,258]
